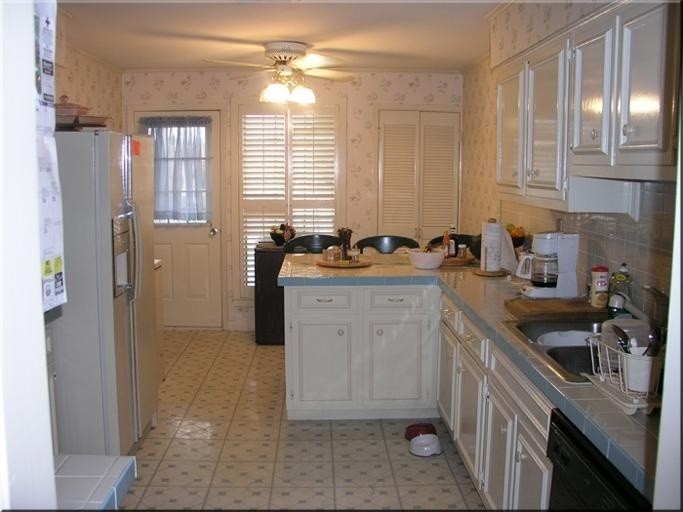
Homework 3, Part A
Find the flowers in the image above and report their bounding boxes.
[272,222,296,242]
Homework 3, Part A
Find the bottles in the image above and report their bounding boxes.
[442,224,466,259]
[607,262,629,299]
[588,264,609,312]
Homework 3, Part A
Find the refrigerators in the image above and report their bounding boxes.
[46,130,160,456]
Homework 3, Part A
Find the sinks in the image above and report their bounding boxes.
[517,316,620,346]
[544,346,605,378]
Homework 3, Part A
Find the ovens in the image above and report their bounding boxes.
[546,408,654,510]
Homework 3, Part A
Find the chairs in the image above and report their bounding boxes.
[286,235,473,254]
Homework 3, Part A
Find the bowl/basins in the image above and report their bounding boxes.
[405,434,442,455]
[269,231,294,246]
[404,423,435,442]
[406,248,444,270]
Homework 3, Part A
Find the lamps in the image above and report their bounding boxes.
[260,69,316,105]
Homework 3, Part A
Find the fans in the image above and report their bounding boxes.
[207,42,355,81]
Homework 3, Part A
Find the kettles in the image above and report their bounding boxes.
[479,217,505,272]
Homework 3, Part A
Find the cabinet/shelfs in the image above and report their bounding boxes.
[490,28,640,224]
[566,0,683,182]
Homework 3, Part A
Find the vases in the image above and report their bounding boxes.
[271,233,284,246]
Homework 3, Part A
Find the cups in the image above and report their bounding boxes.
[622,346,661,393]
[347,248,360,263]
[321,248,335,262]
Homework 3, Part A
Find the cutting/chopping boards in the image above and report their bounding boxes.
[504,298,610,323]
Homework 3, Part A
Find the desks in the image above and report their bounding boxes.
[254,242,285,345]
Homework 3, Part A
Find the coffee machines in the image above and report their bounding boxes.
[513,228,580,299]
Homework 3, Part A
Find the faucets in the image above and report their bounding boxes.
[609,291,648,323]
[640,283,669,325]
